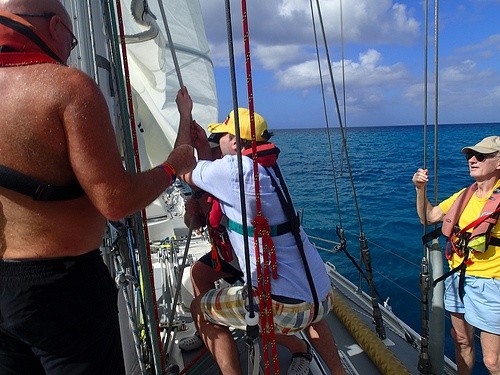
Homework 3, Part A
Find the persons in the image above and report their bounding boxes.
[172,85,333,375]
[177,130,313,375]
[411,135,500,375]
[0,0,197,375]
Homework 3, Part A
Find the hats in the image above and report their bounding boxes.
[207,107,269,141]
[461,136,500,154]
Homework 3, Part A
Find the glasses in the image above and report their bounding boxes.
[45,12,78,51]
[465,150,498,162]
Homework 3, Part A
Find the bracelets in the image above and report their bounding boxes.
[158,162,178,185]
